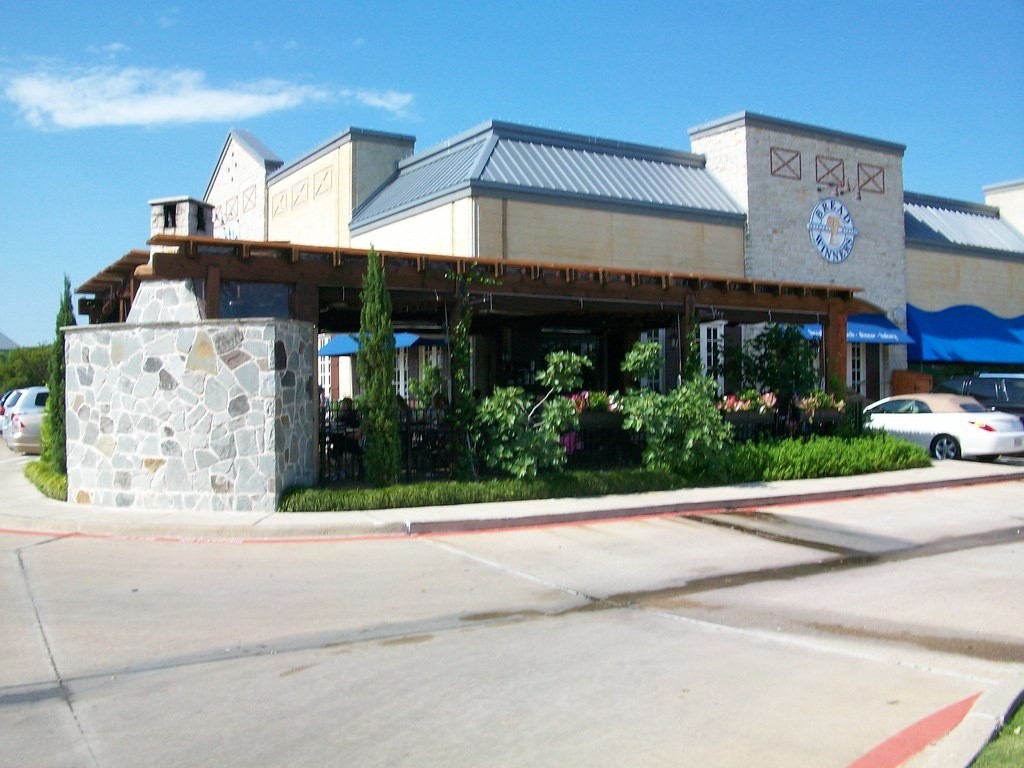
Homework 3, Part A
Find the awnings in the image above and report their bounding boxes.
[318,332,421,356]
[778,312,916,344]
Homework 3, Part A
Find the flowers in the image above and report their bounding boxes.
[801,390,845,424]
[570,391,622,411]
[718,392,777,413]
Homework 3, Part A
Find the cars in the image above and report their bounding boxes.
[862,392,1024,461]
[8,413,46,454]
[3,386,51,440]
[0,389,13,434]
[0,389,25,433]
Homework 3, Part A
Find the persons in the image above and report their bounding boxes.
[318,384,325,407]
[328,393,452,483]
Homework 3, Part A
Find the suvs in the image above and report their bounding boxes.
[929,372,1024,422]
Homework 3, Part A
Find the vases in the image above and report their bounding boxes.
[724,410,775,422]
[801,409,841,422]
[581,412,618,428]
